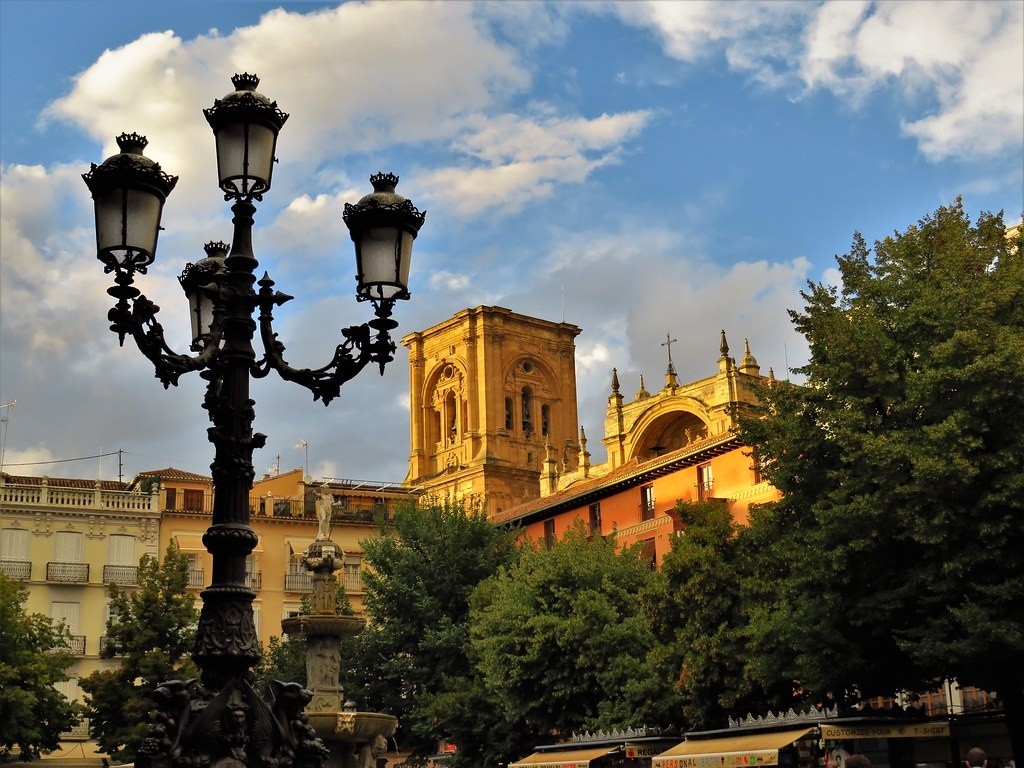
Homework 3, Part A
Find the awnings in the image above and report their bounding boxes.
[507,721,951,768]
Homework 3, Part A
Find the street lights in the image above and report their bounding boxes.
[82,73,427,768]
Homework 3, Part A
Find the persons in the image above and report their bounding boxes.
[845,747,1023,767]
[834,755,842,767]
[317,640,343,685]
[312,484,342,541]
